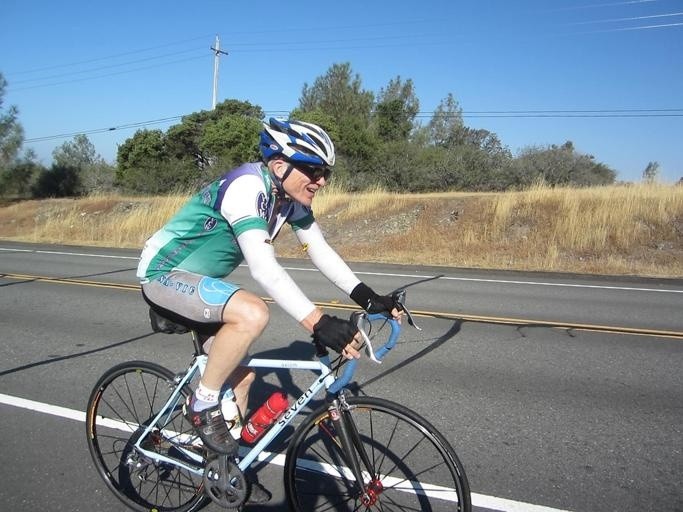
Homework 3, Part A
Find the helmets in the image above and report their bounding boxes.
[258,116,335,168]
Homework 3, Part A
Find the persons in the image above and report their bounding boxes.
[136,116,406,505]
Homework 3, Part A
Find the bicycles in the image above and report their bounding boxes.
[83,271,474,512]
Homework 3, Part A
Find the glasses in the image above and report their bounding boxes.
[281,155,333,182]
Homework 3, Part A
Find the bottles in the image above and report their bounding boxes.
[194,354,242,441]
[239,390,291,443]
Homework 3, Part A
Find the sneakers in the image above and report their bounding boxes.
[181,393,239,455]
[233,480,270,504]
